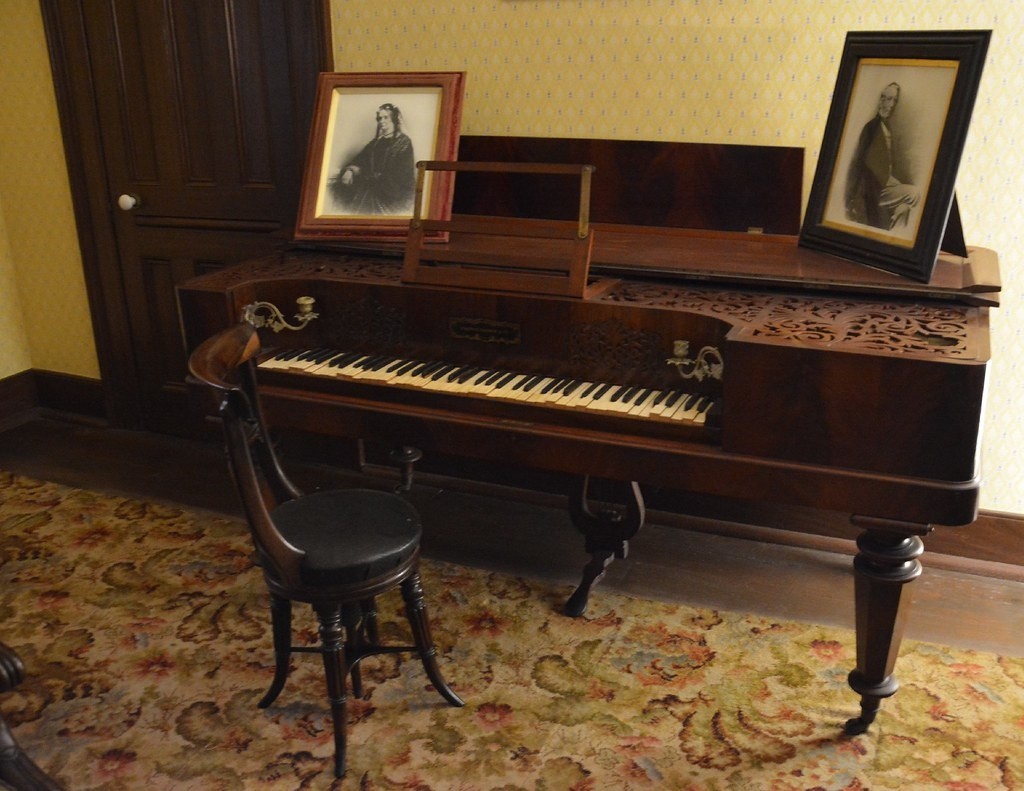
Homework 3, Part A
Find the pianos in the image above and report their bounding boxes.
[170,204,1002,737]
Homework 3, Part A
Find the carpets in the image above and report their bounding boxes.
[0,469,1024,791]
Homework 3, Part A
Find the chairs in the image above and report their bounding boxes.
[186,320,467,777]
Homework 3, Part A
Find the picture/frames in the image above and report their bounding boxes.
[292,71,467,243]
[797,27,994,286]
